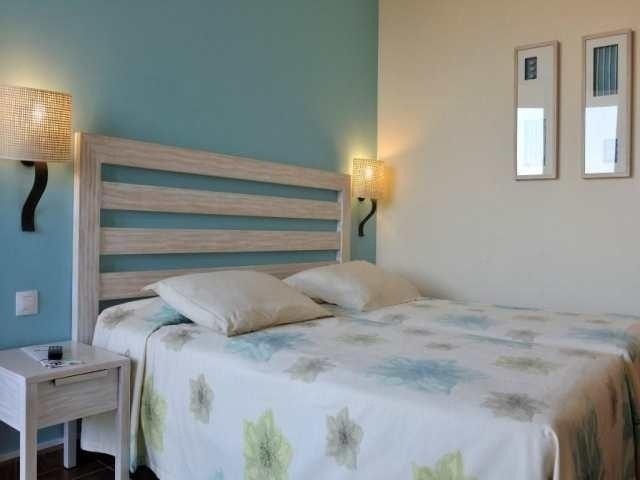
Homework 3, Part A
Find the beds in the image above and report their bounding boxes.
[72,131,640,480]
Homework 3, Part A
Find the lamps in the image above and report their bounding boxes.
[0,85,72,232]
[351,158,389,237]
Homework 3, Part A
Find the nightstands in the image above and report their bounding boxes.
[0,340,130,480]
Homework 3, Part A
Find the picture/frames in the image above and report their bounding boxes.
[512,40,558,181]
[579,28,633,180]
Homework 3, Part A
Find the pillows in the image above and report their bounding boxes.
[141,259,423,337]
